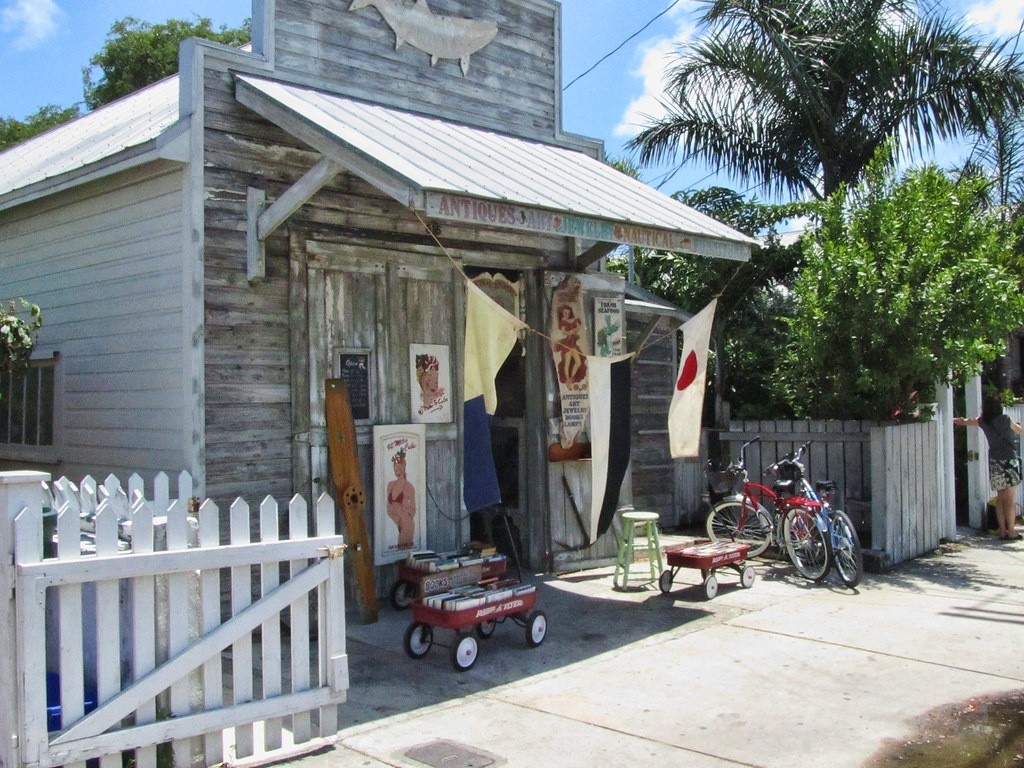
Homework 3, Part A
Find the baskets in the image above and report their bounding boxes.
[704,455,739,493]
[762,452,801,492]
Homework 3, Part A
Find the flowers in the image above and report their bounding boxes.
[0,297,42,373]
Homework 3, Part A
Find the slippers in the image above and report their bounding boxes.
[999,534,1023,541]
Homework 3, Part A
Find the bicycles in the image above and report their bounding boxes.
[761,438,863,588]
[705,436,833,582]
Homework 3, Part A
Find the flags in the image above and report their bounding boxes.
[588,352,634,544]
[667,300,718,458]
[462,279,525,513]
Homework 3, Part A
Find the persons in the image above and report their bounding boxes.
[953,395,1024,541]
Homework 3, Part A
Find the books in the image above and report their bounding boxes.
[404,541,507,575]
[421,576,537,611]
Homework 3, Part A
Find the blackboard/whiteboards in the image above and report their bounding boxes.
[339,353,370,420]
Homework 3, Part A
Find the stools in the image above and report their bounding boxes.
[613,512,665,591]
